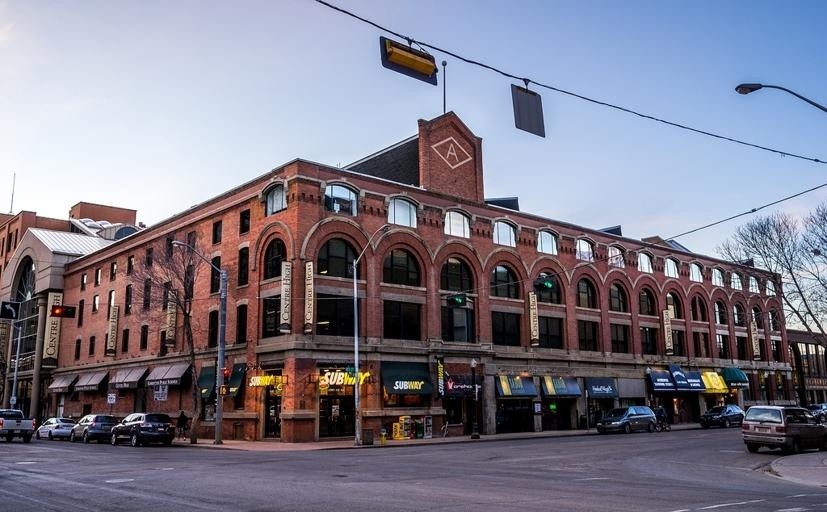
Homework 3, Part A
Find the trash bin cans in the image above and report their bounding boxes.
[234,423,243,438]
[364,429,374,444]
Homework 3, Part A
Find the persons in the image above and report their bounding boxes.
[175,411,187,441]
[654,405,667,432]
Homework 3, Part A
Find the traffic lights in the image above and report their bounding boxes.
[445,294,467,308]
[532,277,555,292]
[224,369,229,385]
[51,305,76,318]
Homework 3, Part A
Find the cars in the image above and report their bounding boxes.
[0,408,176,446]
[700,403,745,428]
[807,404,827,422]
[741,405,827,454]
[596,406,657,434]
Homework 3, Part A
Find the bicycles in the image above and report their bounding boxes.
[656,415,671,432]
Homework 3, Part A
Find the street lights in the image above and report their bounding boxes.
[170,239,228,445]
[1,318,22,408]
[351,223,391,446]
[470,358,479,439]
